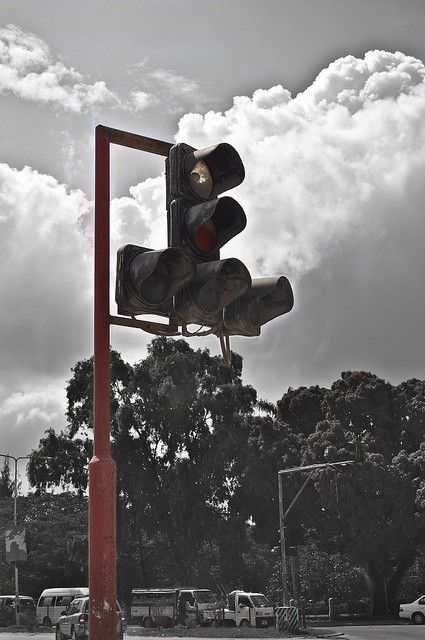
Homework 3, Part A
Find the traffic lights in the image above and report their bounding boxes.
[116,243,293,338]
[166,142,247,263]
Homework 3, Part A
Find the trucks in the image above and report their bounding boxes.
[129,587,222,629]
[219,589,276,629]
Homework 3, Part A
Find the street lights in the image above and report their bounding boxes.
[0,453,52,628]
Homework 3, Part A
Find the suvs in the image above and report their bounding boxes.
[54,596,127,639]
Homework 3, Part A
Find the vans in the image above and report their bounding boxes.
[1,595,33,618]
[34,586,90,631]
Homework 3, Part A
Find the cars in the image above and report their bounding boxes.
[398,594,425,625]
